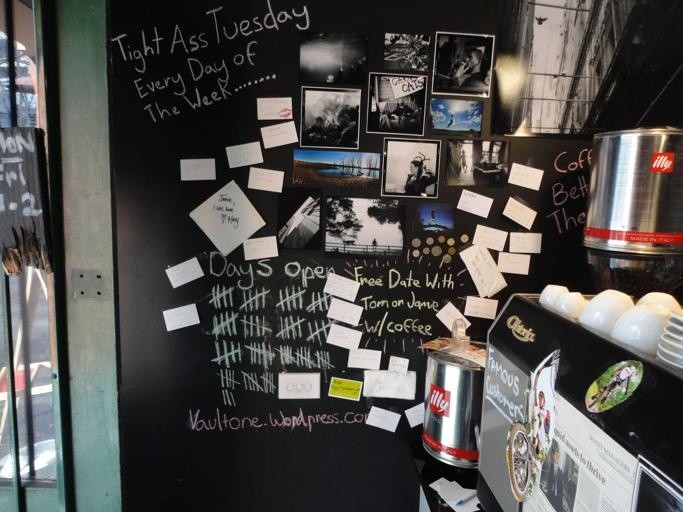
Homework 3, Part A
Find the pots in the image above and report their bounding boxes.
[421,337,487,471]
[579,123,683,272]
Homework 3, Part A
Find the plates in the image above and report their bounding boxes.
[655,313,682,372]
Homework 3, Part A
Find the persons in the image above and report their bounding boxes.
[446,48,483,91]
[372,238,378,252]
[403,152,437,196]
[431,208,437,225]
[389,97,416,120]
[461,149,468,170]
[529,356,555,454]
[326,105,358,147]
[590,364,632,406]
[303,116,330,146]
[434,34,458,90]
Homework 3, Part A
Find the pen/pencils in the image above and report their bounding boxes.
[457,494,483,506]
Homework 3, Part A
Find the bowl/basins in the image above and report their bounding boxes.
[537,282,682,356]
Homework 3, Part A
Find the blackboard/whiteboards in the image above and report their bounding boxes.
[105,6,600,511]
